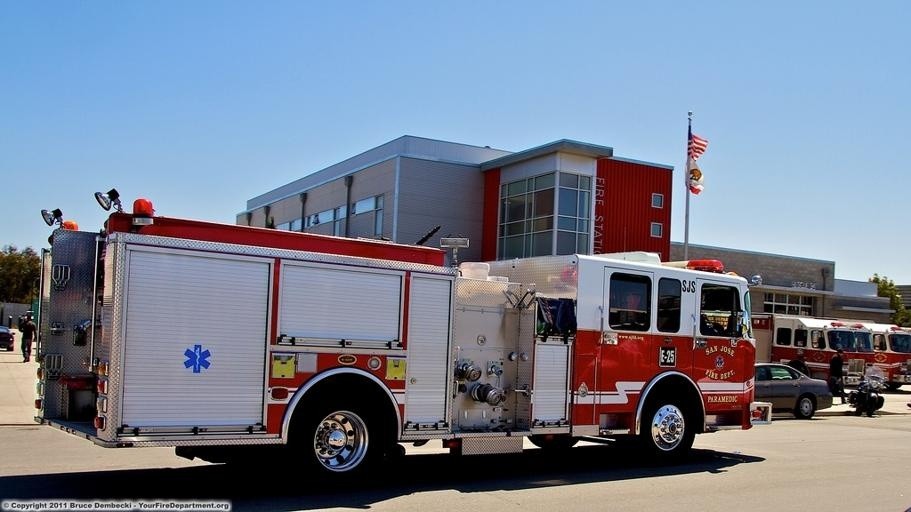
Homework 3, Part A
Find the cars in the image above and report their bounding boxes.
[753,362,833,419]
[0,326,16,350]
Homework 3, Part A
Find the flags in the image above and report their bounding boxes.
[683,121,708,196]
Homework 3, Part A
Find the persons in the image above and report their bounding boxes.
[785,354,810,379]
[825,346,848,406]
[18,313,37,364]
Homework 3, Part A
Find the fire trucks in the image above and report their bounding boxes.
[753,311,911,389]
[35,190,774,490]
